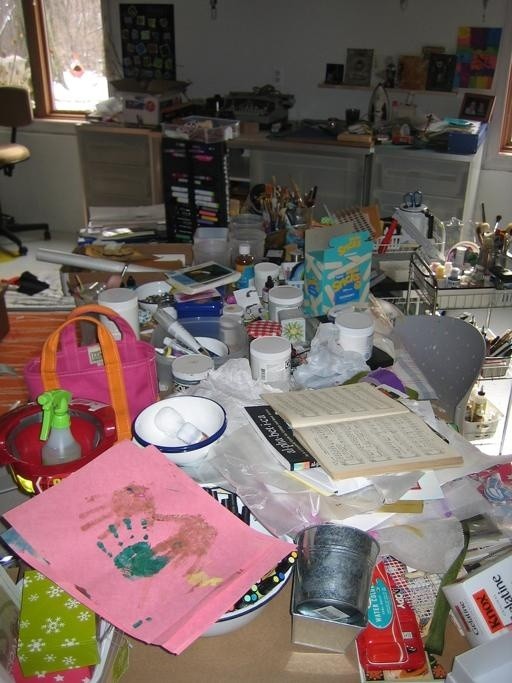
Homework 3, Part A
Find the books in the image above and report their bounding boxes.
[242,382,464,481]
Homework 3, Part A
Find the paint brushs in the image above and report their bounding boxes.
[476,203,512,254]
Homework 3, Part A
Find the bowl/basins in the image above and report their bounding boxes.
[150,315,250,389]
[200,484,298,636]
[135,395,229,465]
[136,279,172,309]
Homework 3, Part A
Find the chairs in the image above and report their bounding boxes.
[1,86,53,256]
[391,314,486,435]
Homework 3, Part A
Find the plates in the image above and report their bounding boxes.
[446,117,471,125]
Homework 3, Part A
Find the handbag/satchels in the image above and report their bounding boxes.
[21,303,162,447]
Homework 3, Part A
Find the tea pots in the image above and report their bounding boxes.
[398,202,433,242]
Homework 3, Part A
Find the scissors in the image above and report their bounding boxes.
[404,191,424,208]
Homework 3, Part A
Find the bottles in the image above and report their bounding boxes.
[429,260,460,288]
[472,386,487,420]
[454,246,491,287]
[158,294,179,319]
[234,246,255,289]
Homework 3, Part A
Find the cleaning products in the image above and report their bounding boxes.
[36,390,81,469]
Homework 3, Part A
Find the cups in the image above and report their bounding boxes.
[230,213,265,230]
[228,230,267,261]
[205,94,224,116]
[345,108,360,123]
[291,200,316,228]
[190,239,232,267]
[73,282,111,344]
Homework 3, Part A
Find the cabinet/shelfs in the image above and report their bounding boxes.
[399,244,512,445]
[76,113,486,258]
[370,208,447,317]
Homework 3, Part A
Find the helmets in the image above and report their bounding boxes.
[0,396,118,499]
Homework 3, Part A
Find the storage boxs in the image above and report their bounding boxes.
[59,239,195,301]
[110,75,194,128]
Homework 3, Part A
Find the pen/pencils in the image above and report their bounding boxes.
[441,310,512,358]
[253,174,318,233]
[202,487,298,614]
[163,142,220,243]
[66,265,136,305]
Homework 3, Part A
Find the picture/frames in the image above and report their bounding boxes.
[341,45,376,89]
[457,91,498,123]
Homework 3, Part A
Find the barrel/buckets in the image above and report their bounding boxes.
[296,524,380,628]
[229,211,264,228]
[230,228,269,265]
